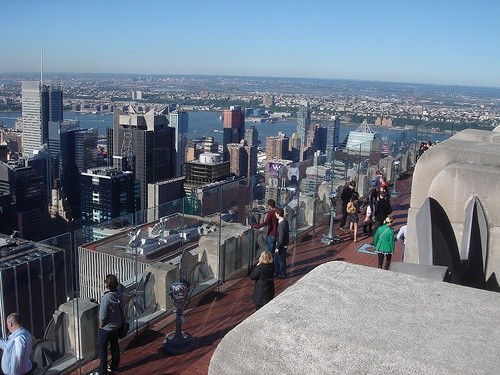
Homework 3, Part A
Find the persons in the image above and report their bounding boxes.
[253,199,279,252]
[390,140,416,154]
[0,312,33,375]
[373,215,396,270]
[396,216,408,262]
[380,186,392,216]
[379,177,391,198]
[288,162,300,189]
[339,180,356,232]
[222,202,240,223]
[346,192,360,243]
[250,250,274,311]
[363,188,378,236]
[419,140,441,155]
[86,273,126,375]
[273,208,289,279]
[370,192,389,246]
[373,170,382,191]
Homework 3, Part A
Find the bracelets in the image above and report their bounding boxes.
[99,326,103,329]
